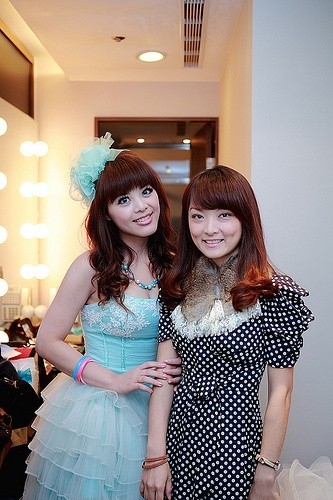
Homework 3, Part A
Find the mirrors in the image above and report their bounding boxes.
[1,98,39,330]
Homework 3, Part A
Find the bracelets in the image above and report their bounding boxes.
[254,454,281,471]
[72,356,97,383]
[142,457,170,470]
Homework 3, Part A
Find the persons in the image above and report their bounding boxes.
[140,166,315,500]
[23,132,183,500]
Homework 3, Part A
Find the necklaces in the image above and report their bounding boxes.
[120,263,158,290]
